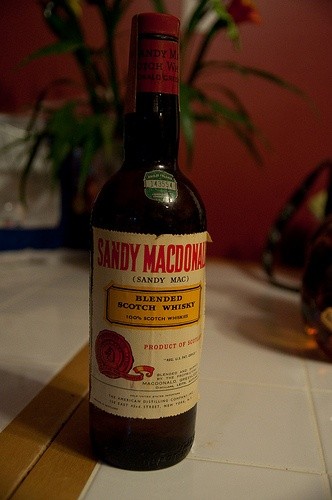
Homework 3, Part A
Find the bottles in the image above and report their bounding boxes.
[87,11,208,472]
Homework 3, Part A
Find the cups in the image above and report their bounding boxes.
[300,219,332,363]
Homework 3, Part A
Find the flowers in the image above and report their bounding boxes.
[3,1,311,218]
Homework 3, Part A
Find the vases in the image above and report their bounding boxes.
[56,131,128,248]
